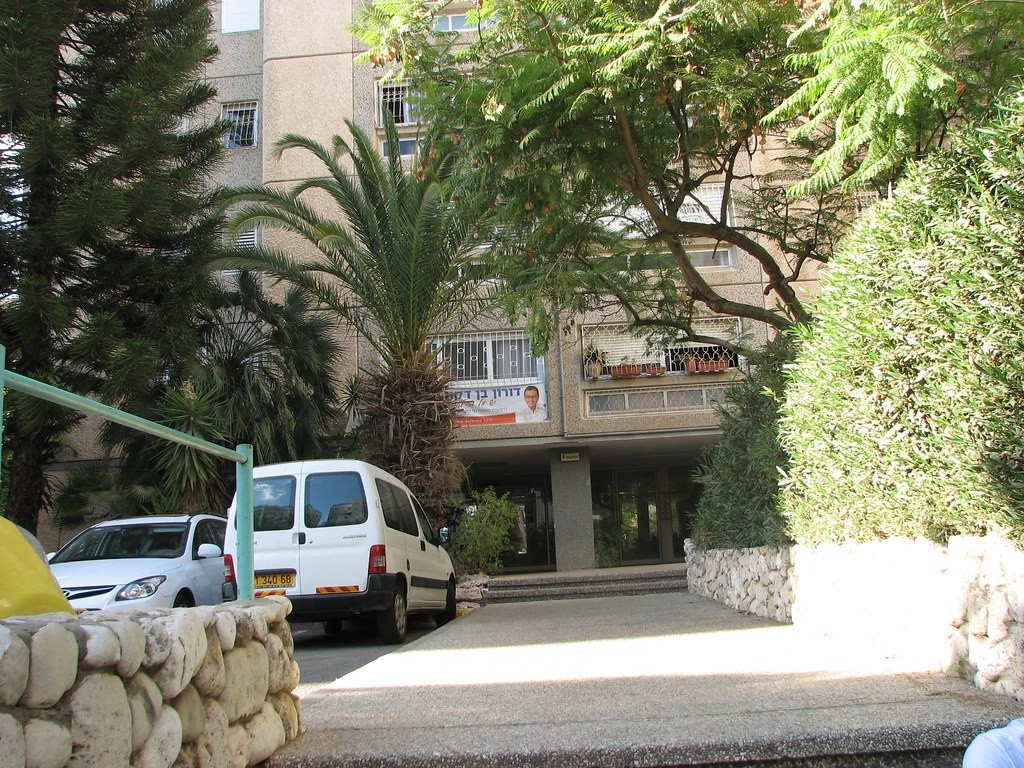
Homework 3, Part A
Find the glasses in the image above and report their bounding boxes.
[525,396,538,399]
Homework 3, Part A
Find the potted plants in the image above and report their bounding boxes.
[645,362,666,377]
[679,353,731,374]
[611,355,642,377]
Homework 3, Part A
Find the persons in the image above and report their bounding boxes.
[515,386,546,422]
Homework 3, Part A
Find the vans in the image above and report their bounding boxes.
[219,457,457,644]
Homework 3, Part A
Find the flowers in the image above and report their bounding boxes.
[583,337,608,367]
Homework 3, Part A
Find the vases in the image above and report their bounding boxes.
[586,360,602,379]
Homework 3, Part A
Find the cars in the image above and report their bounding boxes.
[44,515,230,616]
